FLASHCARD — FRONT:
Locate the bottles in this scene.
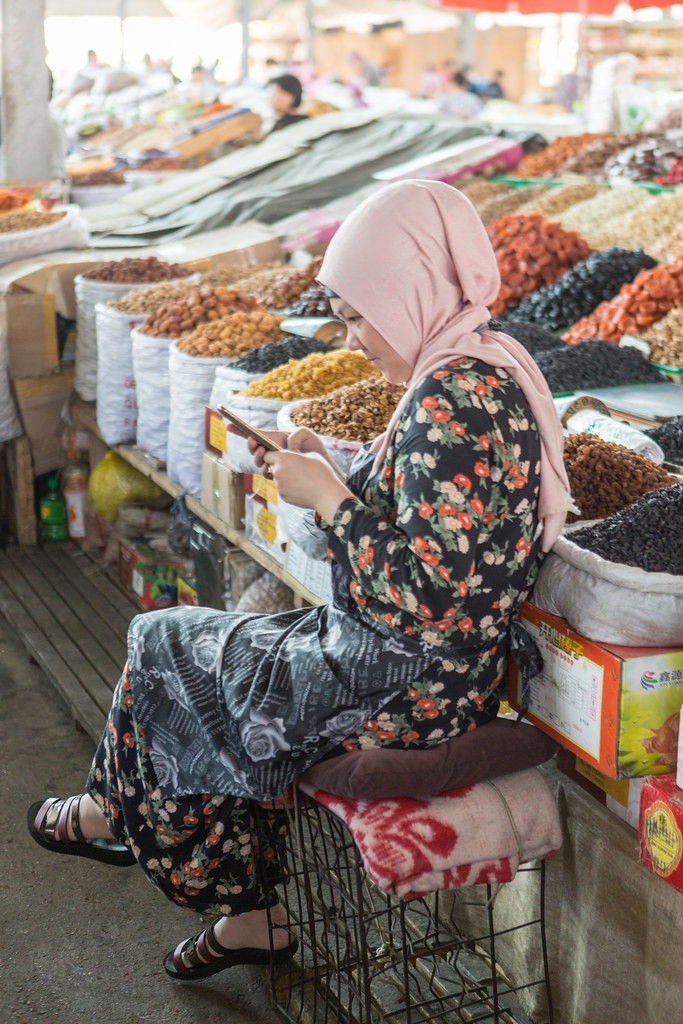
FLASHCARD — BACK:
[40,478,68,543]
[63,451,88,541]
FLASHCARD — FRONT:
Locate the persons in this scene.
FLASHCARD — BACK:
[347,49,389,88]
[24,178,581,980]
[262,73,308,132]
[420,58,505,102]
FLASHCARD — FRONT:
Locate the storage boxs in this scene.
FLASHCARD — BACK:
[6,293,60,379]
[176,575,199,607]
[117,536,183,610]
[10,325,83,477]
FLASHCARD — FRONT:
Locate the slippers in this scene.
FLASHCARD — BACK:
[163,915,299,980]
[27,793,138,867]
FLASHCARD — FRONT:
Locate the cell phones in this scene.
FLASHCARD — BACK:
[217,404,282,453]
